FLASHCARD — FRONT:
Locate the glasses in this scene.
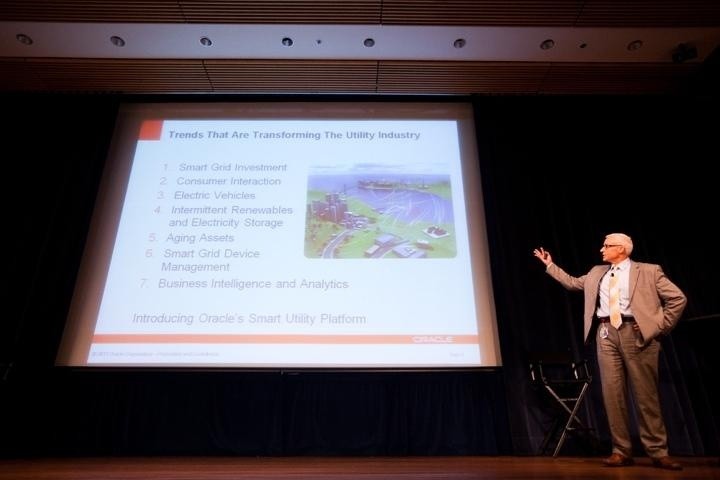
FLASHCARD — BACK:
[602,244,624,249]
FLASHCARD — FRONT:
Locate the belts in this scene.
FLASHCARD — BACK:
[598,318,633,323]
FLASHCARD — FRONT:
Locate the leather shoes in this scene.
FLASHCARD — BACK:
[601,454,633,467]
[653,456,683,470]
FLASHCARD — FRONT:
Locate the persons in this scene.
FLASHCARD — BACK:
[530,230,689,471]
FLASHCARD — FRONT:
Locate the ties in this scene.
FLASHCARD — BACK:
[609,267,623,330]
